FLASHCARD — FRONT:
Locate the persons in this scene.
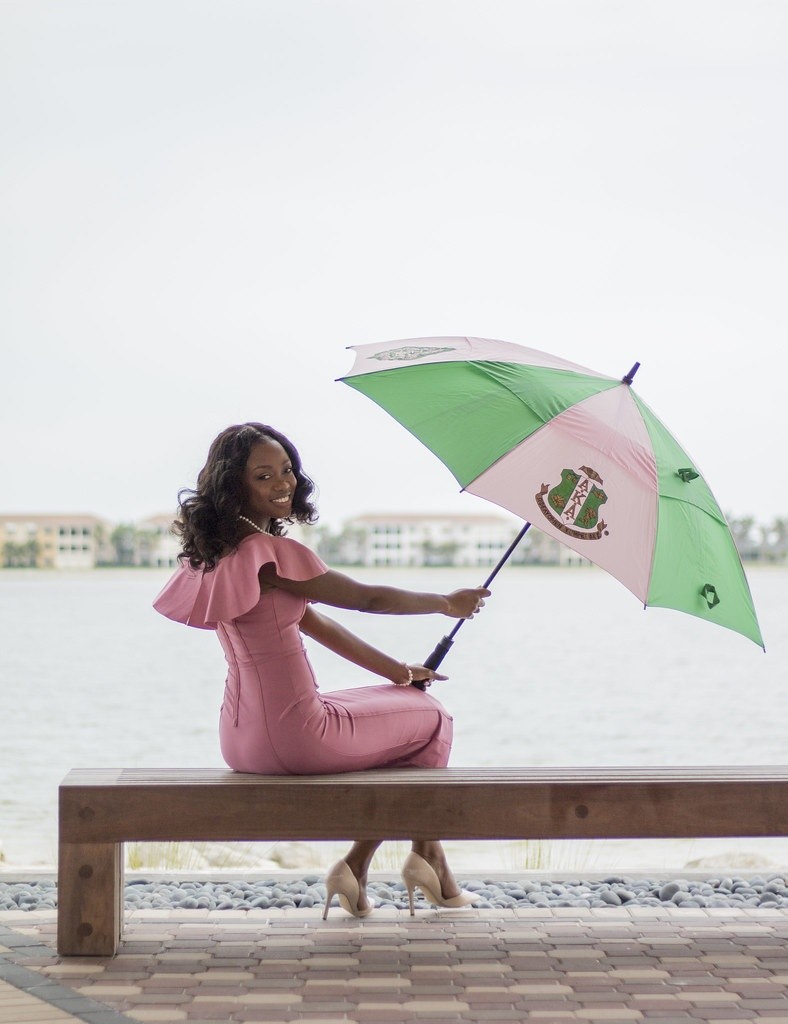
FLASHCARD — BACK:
[151,420,492,925]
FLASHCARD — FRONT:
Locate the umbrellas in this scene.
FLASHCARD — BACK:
[315,334,768,693]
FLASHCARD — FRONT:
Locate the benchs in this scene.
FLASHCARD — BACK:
[57,766,788,957]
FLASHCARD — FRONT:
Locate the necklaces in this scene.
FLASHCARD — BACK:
[239,515,274,536]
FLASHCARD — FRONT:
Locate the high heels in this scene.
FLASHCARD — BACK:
[323,858,376,920]
[402,850,480,916]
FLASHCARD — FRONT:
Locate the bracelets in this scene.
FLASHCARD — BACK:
[393,661,414,686]
